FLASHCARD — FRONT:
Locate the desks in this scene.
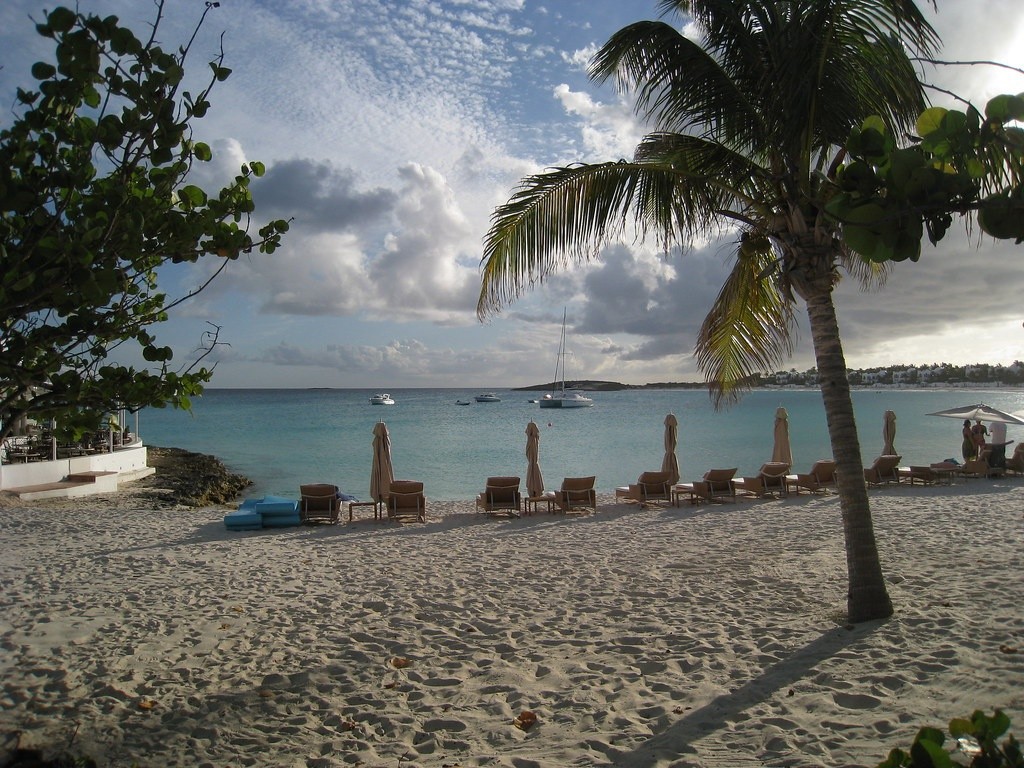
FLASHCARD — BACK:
[898,467,911,485]
[10,453,40,463]
[786,474,798,494]
[731,478,746,496]
[523,494,556,516]
[348,501,377,522]
[672,484,693,508]
[64,448,95,456]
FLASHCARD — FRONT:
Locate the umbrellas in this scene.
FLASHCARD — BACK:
[661,412,680,503]
[772,402,793,492]
[370,420,394,521]
[925,402,1024,425]
[525,417,544,512]
[883,410,897,455]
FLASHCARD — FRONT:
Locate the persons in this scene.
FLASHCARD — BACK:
[962,420,1007,468]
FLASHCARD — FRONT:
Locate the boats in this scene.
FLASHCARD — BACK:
[455,400,471,405]
[371,393,395,405]
[528,399,539,403]
[474,393,502,402]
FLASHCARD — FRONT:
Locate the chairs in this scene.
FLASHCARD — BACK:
[616,472,671,509]
[864,455,902,490]
[547,475,598,513]
[476,476,522,519]
[0,436,51,464]
[387,479,427,523]
[742,463,790,500]
[909,463,931,487]
[796,459,838,496]
[693,468,739,508]
[967,443,1024,479]
[931,460,959,483]
[224,494,301,532]
[300,483,341,526]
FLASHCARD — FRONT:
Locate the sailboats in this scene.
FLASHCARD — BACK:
[540,306,592,409]
